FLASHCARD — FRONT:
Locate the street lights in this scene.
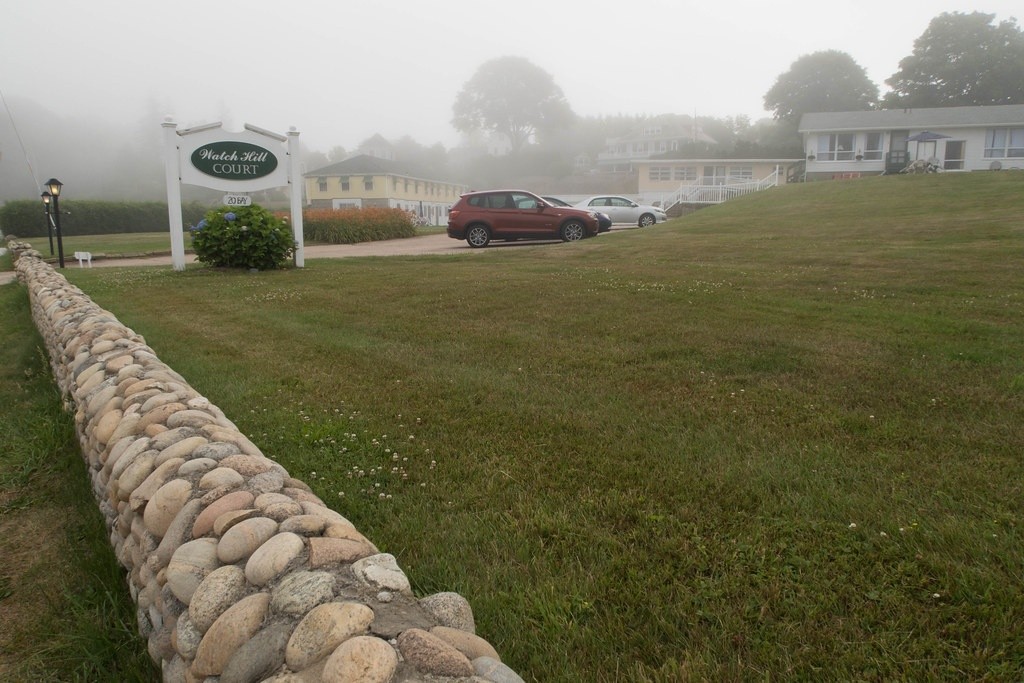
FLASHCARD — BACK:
[43,177,65,268]
[41,191,55,257]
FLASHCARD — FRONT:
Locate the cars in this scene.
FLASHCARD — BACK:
[496,196,613,243]
[571,196,668,227]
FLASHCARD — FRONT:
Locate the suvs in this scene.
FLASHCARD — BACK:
[445,189,600,249]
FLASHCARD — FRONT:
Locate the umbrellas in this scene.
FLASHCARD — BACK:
[906,130,951,162]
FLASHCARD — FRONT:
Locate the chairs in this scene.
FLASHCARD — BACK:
[899,157,944,175]
